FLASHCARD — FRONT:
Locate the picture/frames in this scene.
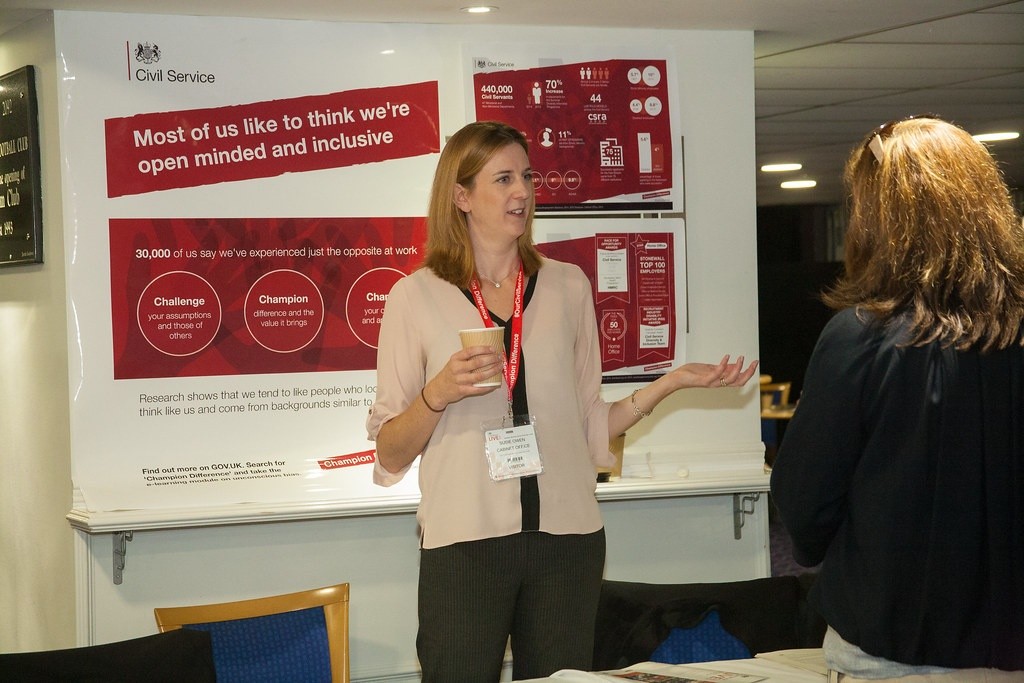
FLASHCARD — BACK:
[0,64,42,268]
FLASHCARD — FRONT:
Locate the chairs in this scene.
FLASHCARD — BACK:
[592,574,827,671]
[155,583,350,683]
[0,629,216,683]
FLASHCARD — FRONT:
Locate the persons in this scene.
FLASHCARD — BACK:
[366,121,759,683]
[771,260,841,450]
[770,115,1023,678]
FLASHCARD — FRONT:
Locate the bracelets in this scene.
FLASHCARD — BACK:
[421,387,447,412]
[632,388,653,417]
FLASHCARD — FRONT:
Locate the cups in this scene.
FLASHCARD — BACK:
[458,326,505,388]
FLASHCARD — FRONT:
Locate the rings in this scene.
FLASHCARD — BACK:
[720,378,726,385]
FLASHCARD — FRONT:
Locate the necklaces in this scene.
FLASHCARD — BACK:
[478,259,519,288]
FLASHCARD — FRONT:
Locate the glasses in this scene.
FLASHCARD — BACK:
[863,114,934,166]
